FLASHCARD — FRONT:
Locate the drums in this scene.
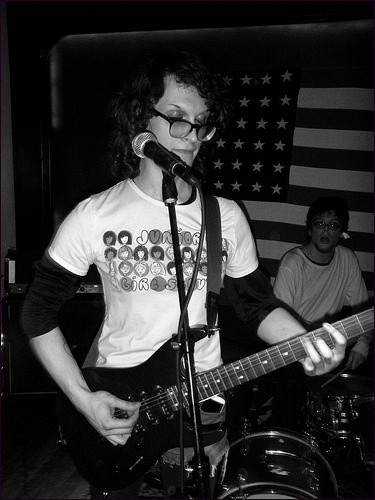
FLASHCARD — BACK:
[302,374,375,439]
[218,432,339,500]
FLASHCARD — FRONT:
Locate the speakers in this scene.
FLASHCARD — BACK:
[6,294,105,395]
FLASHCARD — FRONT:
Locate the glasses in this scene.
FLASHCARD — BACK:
[154,109,216,142]
[312,220,341,230]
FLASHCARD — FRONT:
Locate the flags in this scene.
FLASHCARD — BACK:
[197,65,375,292]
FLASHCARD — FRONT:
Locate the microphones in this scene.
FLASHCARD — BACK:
[131,131,201,188]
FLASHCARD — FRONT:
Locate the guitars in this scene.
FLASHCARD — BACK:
[55,306,375,491]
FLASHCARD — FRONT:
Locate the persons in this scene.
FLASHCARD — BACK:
[21,52,346,499]
[273,197,374,371]
